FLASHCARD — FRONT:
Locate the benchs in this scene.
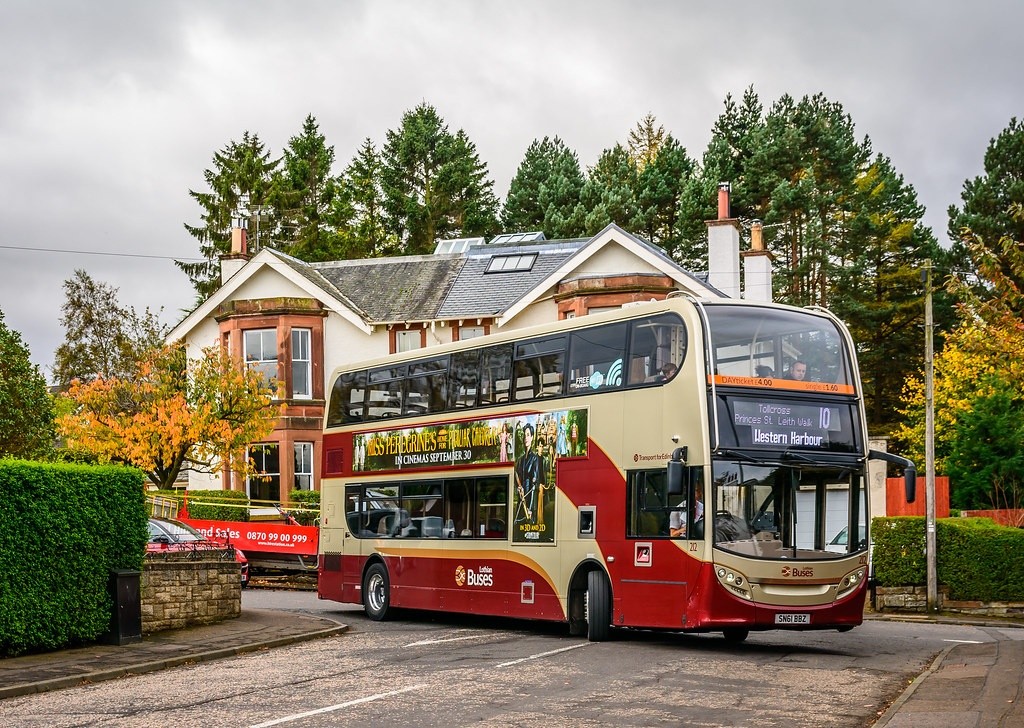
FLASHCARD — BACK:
[347,510,396,537]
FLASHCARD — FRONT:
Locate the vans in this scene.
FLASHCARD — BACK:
[349,490,416,537]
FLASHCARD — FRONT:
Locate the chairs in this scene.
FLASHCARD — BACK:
[535,391,559,399]
[381,412,400,418]
[479,518,505,538]
[450,403,468,409]
[377,515,395,538]
[472,400,493,407]
[422,517,444,538]
[498,397,518,403]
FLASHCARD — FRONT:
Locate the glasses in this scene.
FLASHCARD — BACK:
[695,489,701,492]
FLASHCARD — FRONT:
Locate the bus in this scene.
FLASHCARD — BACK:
[316,296,919,641]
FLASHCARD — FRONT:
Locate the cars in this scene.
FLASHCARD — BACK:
[145,516,249,590]
[458,518,506,537]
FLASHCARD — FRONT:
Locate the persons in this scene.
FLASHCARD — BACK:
[756,365,774,380]
[783,361,806,382]
[516,423,546,533]
[668,479,705,538]
[662,363,677,382]
